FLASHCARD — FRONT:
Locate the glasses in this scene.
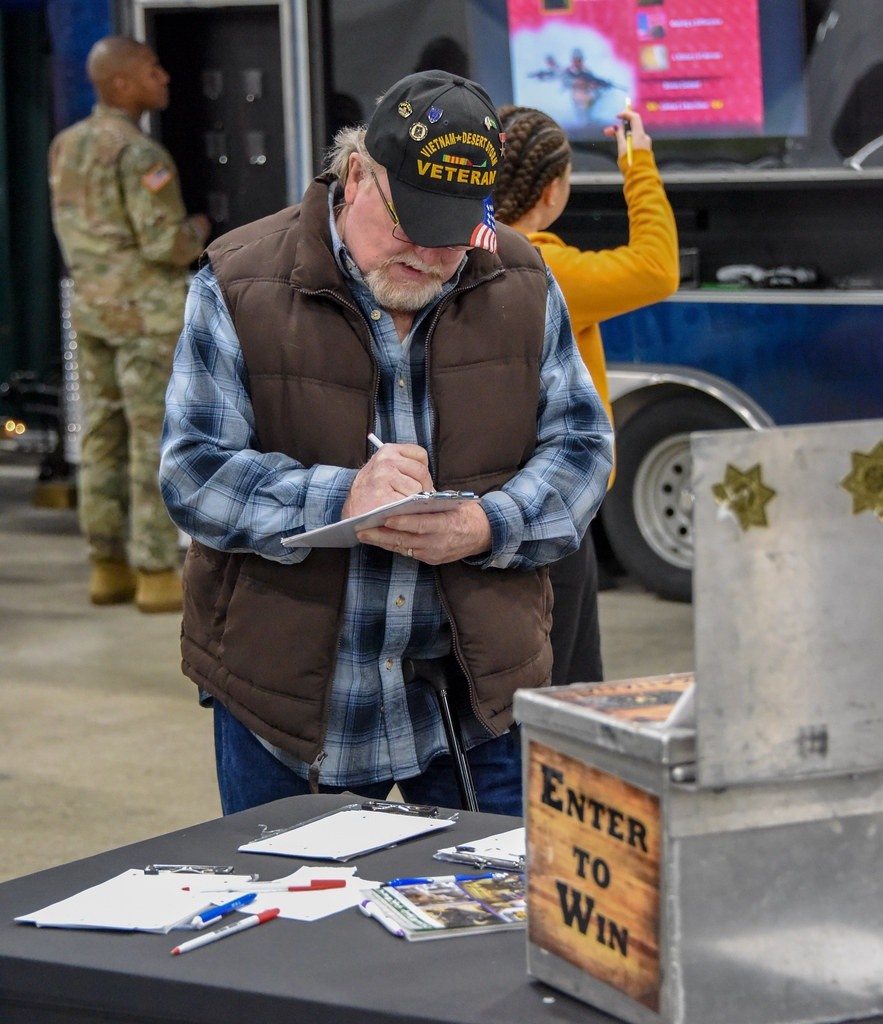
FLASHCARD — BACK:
[369,171,475,252]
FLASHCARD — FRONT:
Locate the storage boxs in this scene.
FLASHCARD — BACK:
[512,413,883,1024]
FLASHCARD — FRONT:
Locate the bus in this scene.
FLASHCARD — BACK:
[0,1,883,609]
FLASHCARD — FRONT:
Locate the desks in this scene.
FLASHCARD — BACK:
[1,793,883,1023]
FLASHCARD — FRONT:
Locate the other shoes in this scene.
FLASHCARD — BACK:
[135,567,184,614]
[89,551,136,605]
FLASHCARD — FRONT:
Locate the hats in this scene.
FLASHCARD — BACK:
[364,69,505,254]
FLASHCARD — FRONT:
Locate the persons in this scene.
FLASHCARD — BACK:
[486,104,679,688]
[157,68,615,819]
[47,35,210,612]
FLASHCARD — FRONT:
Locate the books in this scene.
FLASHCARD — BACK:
[362,871,527,942]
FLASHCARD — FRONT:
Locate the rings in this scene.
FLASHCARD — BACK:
[407,548,413,558]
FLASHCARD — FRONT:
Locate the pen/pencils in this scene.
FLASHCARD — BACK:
[623,96,636,167]
[169,878,347,957]
[358,871,494,937]
[367,431,437,493]
